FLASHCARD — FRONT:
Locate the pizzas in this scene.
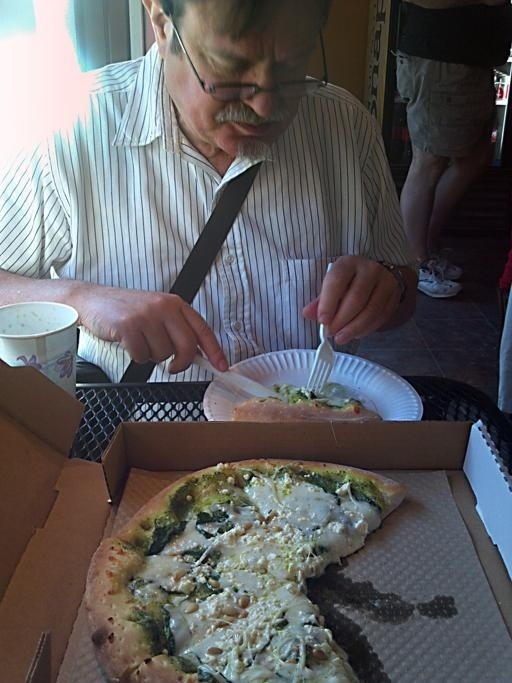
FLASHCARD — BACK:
[230,388,382,423]
[86,458,408,683]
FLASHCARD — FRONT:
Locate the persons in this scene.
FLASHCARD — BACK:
[391,2,510,300]
[2,0,422,421]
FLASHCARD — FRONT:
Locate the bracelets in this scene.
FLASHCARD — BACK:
[378,257,408,307]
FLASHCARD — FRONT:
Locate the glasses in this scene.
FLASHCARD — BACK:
[158,6,328,101]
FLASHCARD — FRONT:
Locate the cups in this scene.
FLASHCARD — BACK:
[0,302,79,399]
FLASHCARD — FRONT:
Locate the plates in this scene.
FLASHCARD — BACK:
[201,348,426,422]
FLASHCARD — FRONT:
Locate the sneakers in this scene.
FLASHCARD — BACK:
[417,262,462,300]
[432,255,463,282]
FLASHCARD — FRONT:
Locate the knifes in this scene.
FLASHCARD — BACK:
[190,351,291,404]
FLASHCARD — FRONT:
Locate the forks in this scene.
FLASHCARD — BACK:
[304,263,335,398]
